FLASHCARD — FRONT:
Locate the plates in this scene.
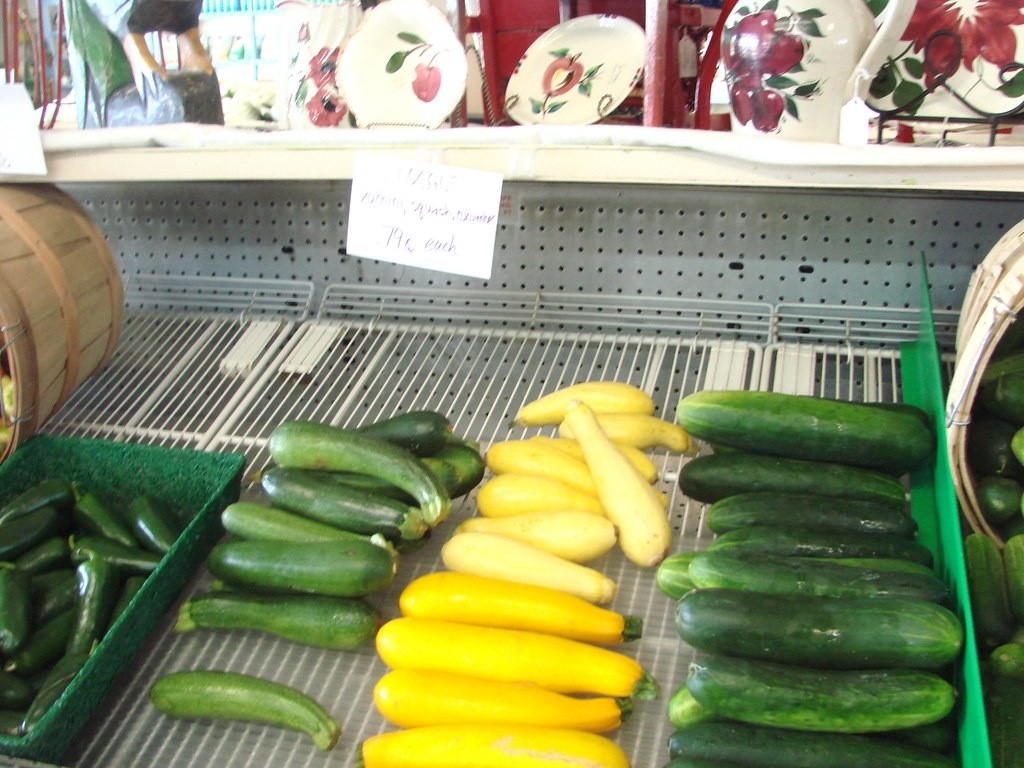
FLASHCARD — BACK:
[505,13,647,127]
[866,0,1024,120]
[335,0,467,128]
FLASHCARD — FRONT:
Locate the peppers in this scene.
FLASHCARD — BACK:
[0,478,180,738]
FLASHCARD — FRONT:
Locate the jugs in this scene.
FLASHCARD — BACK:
[720,0,918,147]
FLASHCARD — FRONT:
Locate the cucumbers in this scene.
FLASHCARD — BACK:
[656,308,1024,768]
